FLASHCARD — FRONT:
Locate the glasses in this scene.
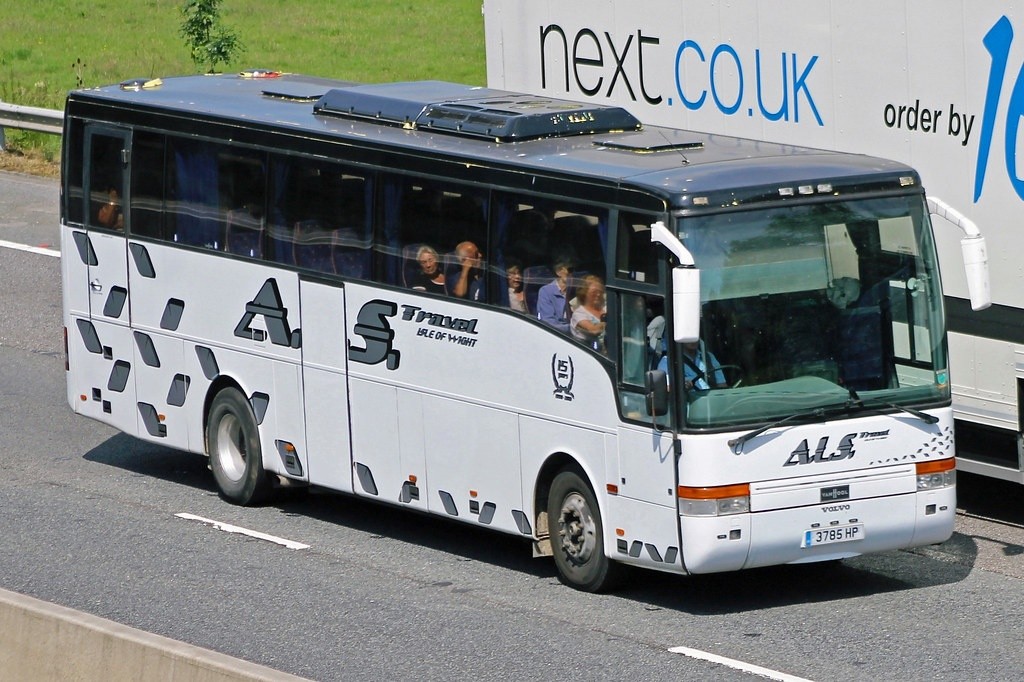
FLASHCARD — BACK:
[508,270,521,275]
[468,250,483,259]
[567,266,582,273]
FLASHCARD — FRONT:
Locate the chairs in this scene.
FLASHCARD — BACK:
[151,183,665,385]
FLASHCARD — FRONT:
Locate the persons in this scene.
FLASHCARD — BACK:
[96,179,124,232]
[653,337,727,394]
[445,239,486,303]
[504,256,528,313]
[406,244,446,294]
[537,253,582,336]
[569,274,608,358]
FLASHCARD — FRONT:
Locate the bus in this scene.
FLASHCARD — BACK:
[61,63,959,591]
